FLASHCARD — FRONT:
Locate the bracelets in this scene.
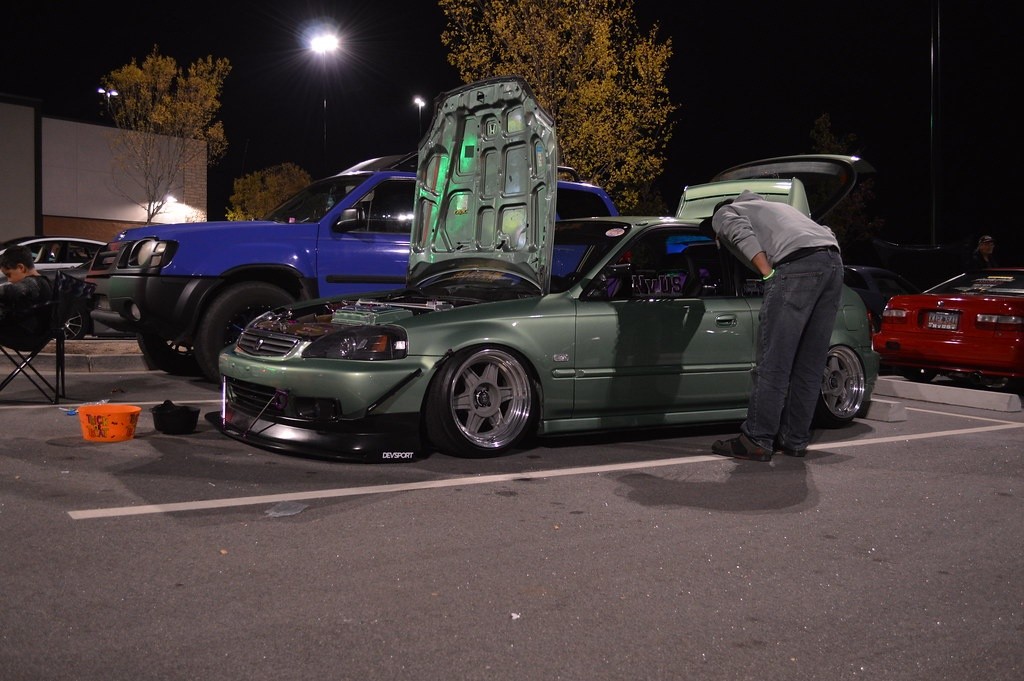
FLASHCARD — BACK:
[763,269,775,280]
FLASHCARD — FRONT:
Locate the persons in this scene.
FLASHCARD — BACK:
[974,235,996,269]
[711,190,844,461]
[0,245,53,309]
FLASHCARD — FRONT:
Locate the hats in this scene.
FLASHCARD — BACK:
[977,235,993,250]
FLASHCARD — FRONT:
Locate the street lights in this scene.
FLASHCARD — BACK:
[310,33,343,177]
[414,96,429,136]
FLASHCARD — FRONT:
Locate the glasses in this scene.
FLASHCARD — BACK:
[983,242,995,247]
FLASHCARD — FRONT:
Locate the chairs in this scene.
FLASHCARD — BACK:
[0,268,99,405]
[640,252,703,300]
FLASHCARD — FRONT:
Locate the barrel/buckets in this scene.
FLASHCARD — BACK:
[148,399,200,435]
[78,404,142,442]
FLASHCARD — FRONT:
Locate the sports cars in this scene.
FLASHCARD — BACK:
[872,268,1024,390]
[214,74,886,458]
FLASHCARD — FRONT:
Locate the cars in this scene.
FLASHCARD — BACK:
[842,263,921,324]
[0,236,108,339]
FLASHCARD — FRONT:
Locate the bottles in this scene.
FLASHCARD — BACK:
[327,198,334,209]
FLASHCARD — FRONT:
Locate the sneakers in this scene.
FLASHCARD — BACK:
[711,433,772,462]
[773,436,806,457]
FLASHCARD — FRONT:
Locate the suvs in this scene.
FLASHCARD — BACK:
[86,149,624,386]
